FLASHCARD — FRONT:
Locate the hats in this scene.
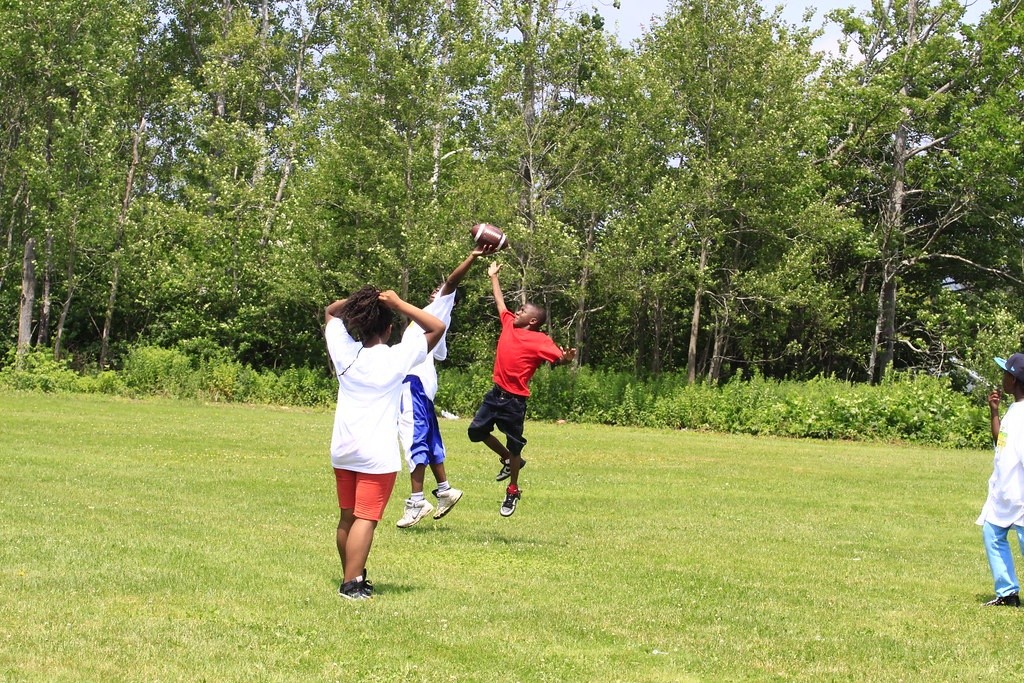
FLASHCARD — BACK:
[994,353,1024,382]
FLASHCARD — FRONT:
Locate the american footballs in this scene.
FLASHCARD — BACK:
[469,222,510,252]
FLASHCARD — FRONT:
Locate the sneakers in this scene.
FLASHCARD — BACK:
[432,487,463,520]
[338,568,377,602]
[496,452,526,481]
[500,488,521,517]
[396,498,435,528]
[981,594,1020,607]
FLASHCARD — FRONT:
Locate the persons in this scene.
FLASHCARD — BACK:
[324,285,447,600]
[981,354,1024,607]
[468,259,577,517]
[396,244,498,528]
